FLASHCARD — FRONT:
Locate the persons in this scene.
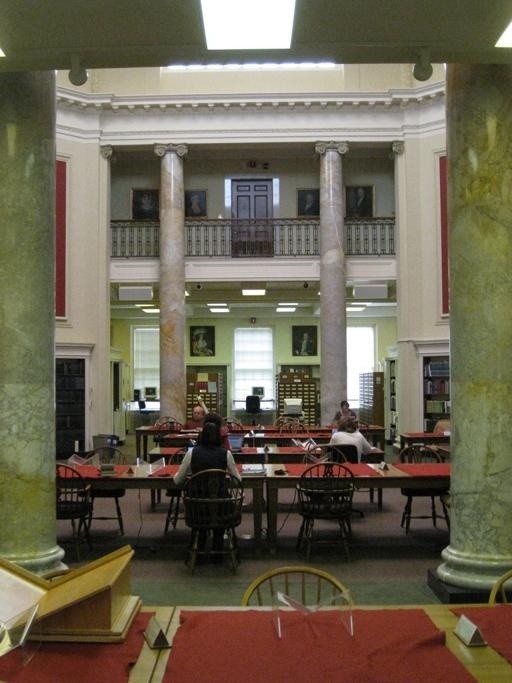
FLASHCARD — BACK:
[191,329,213,355]
[184,405,206,428]
[299,192,319,215]
[171,421,242,566]
[136,190,157,218]
[294,332,312,356]
[328,416,371,465]
[348,186,372,216]
[186,194,205,217]
[202,414,233,452]
[332,400,357,425]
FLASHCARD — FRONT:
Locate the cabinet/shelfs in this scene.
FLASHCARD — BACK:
[383,336,449,444]
[359,373,385,426]
[186,372,223,418]
[55,344,90,460]
[277,378,315,425]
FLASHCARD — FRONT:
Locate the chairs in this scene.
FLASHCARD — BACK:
[241,564,352,606]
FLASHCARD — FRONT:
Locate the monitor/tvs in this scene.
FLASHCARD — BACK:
[283,399,303,415]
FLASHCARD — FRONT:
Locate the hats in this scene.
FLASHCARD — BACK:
[339,416,355,426]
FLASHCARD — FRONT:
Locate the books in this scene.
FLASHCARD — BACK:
[390,360,396,439]
[423,355,451,433]
[275,365,319,424]
[100,462,116,476]
[186,371,223,420]
[240,464,267,478]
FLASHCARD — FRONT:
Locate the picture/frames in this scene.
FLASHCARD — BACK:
[129,186,208,221]
[189,325,216,357]
[291,324,319,357]
[296,185,376,220]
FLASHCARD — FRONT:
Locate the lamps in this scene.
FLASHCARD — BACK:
[412,49,434,81]
[68,54,87,84]
